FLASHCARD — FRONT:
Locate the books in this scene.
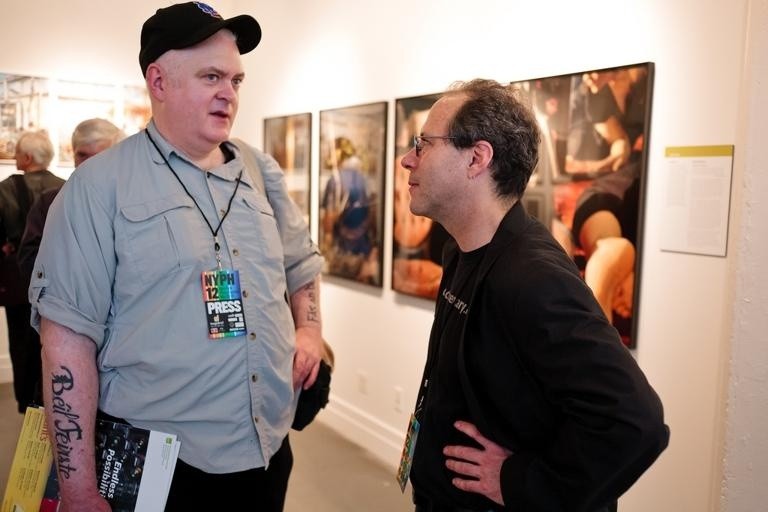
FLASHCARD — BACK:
[0,406,181,511]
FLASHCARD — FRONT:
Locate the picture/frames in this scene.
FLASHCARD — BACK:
[317,100,388,293]
[387,86,475,300]
[261,112,314,246]
[504,60,658,352]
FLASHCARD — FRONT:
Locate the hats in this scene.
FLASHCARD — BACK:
[139,1,261,80]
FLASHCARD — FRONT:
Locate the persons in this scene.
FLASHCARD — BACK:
[16,118,120,285]
[0,129,67,414]
[401,78,670,512]
[27,1,332,512]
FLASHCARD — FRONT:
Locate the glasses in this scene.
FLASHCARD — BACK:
[414,136,460,156]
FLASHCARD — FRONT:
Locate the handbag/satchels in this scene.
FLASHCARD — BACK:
[292,333,335,431]
